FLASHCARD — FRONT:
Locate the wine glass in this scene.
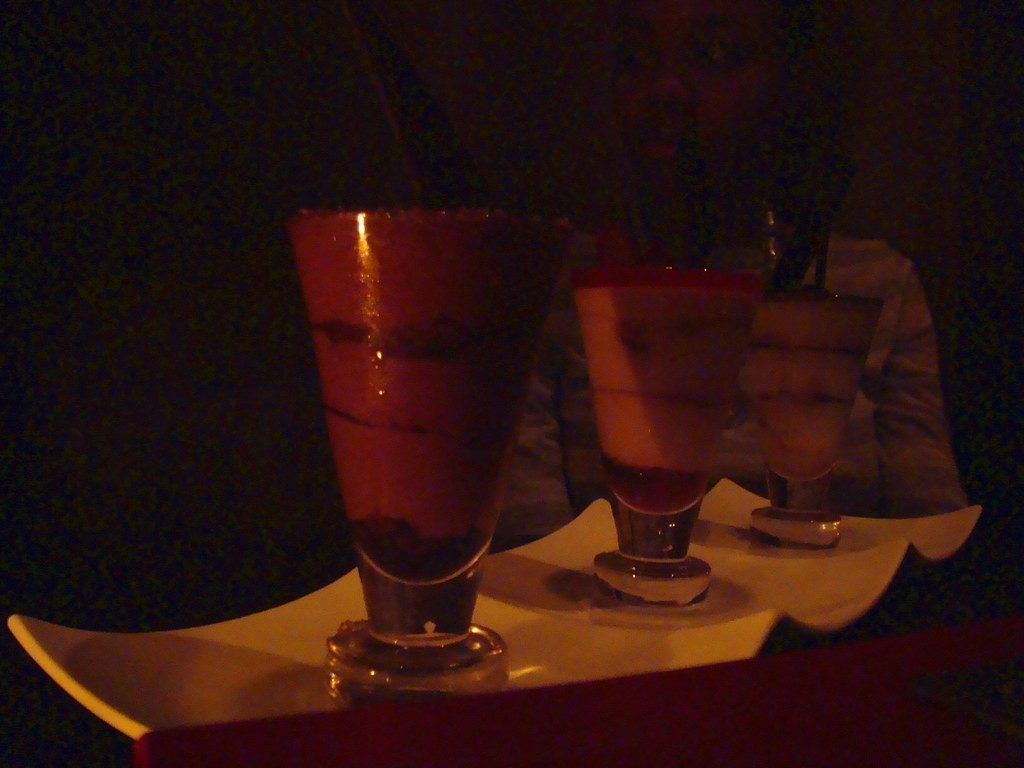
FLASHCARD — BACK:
[746,289,882,544]
[287,203,575,678]
[563,264,757,609]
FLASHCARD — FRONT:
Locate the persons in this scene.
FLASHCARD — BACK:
[494,0,967,546]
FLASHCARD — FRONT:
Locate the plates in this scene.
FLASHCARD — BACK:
[132,614,1024,768]
[4,476,980,740]
